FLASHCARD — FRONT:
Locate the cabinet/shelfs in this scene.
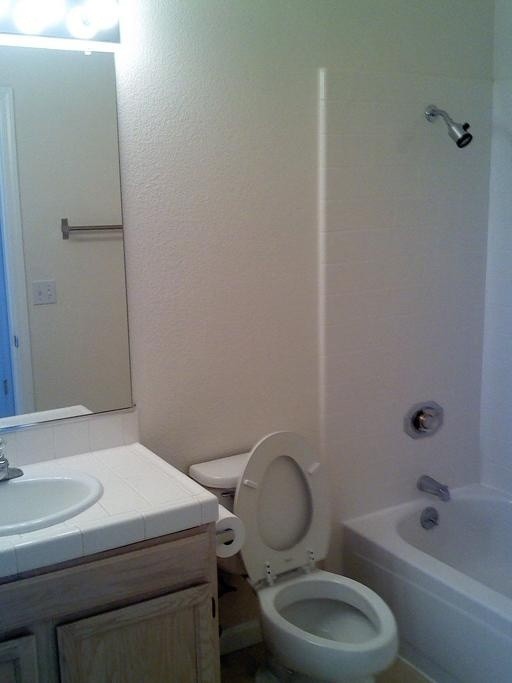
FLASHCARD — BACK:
[0,522,222,683]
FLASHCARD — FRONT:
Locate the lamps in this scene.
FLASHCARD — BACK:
[67,0,117,38]
[8,0,65,33]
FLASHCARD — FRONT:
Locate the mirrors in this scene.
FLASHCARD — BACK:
[0,44,137,436]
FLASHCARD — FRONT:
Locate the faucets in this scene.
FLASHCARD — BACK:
[417,473,453,503]
[1,436,24,483]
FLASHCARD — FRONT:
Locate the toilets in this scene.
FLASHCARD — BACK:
[186,429,399,681]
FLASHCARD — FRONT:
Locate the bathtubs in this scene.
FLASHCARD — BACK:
[342,482,511,680]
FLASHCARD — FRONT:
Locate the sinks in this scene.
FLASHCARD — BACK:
[1,472,105,538]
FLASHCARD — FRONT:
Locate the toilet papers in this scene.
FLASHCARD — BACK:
[213,503,248,561]
